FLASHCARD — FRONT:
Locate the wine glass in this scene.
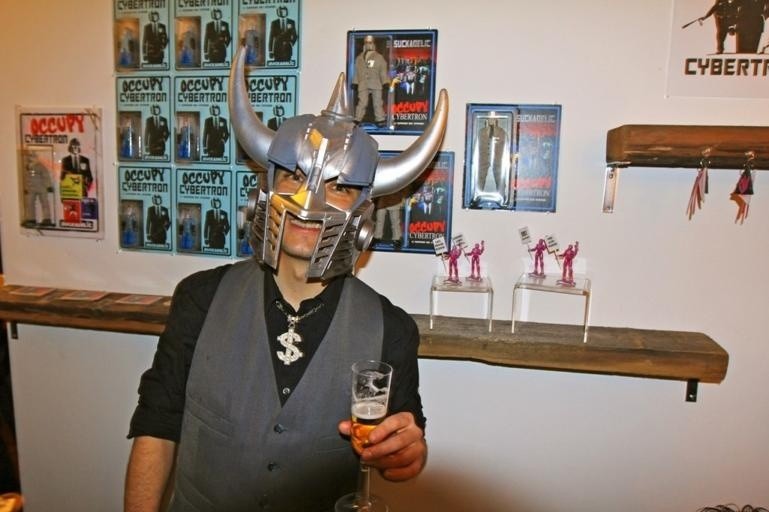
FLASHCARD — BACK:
[333,359,395,512]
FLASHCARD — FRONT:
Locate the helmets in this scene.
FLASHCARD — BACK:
[248,110,382,285]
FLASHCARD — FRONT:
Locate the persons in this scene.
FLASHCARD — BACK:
[59,135,93,200]
[410,180,446,249]
[444,242,463,283]
[464,240,486,282]
[526,238,548,277]
[23,147,56,229]
[351,34,393,128]
[372,189,404,247]
[556,240,582,286]
[124,112,429,511]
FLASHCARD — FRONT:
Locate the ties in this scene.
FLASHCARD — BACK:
[215,209,219,224]
[74,155,79,174]
[216,20,219,33]
[155,116,159,129]
[153,22,157,36]
[156,205,160,217]
[214,117,219,131]
[281,18,286,34]
[277,117,282,130]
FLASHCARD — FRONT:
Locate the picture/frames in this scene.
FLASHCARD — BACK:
[462,103,559,212]
[345,29,436,136]
[367,151,453,255]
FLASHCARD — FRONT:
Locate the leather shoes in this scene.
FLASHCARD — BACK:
[368,238,379,248]
[22,219,51,226]
[393,239,401,250]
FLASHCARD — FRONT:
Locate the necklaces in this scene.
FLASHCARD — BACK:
[271,299,328,366]
